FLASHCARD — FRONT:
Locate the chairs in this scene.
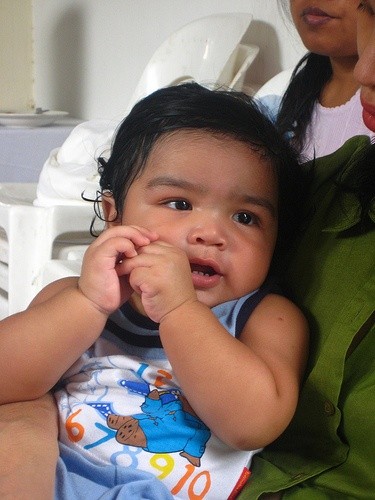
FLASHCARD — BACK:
[0,12,260,318]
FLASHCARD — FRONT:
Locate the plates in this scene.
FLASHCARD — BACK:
[0,111,69,128]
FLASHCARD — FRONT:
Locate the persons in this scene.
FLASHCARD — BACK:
[0,83,308,500]
[241,0,375,500]
[254,0,375,163]
